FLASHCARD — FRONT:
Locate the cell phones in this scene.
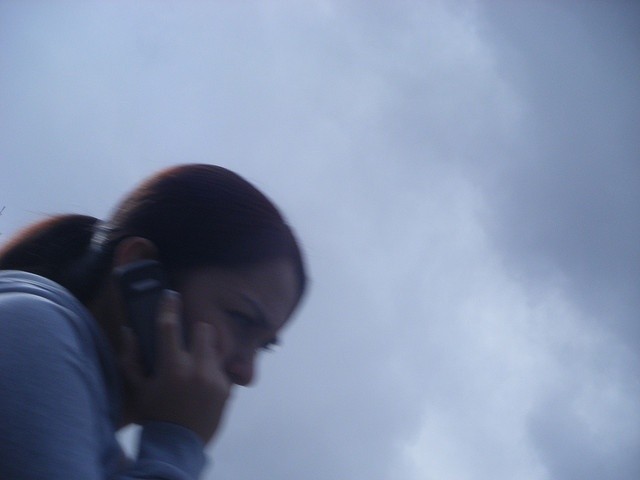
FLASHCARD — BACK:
[110,260,188,380]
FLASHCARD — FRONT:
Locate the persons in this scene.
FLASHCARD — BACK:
[0,162,309,480]
